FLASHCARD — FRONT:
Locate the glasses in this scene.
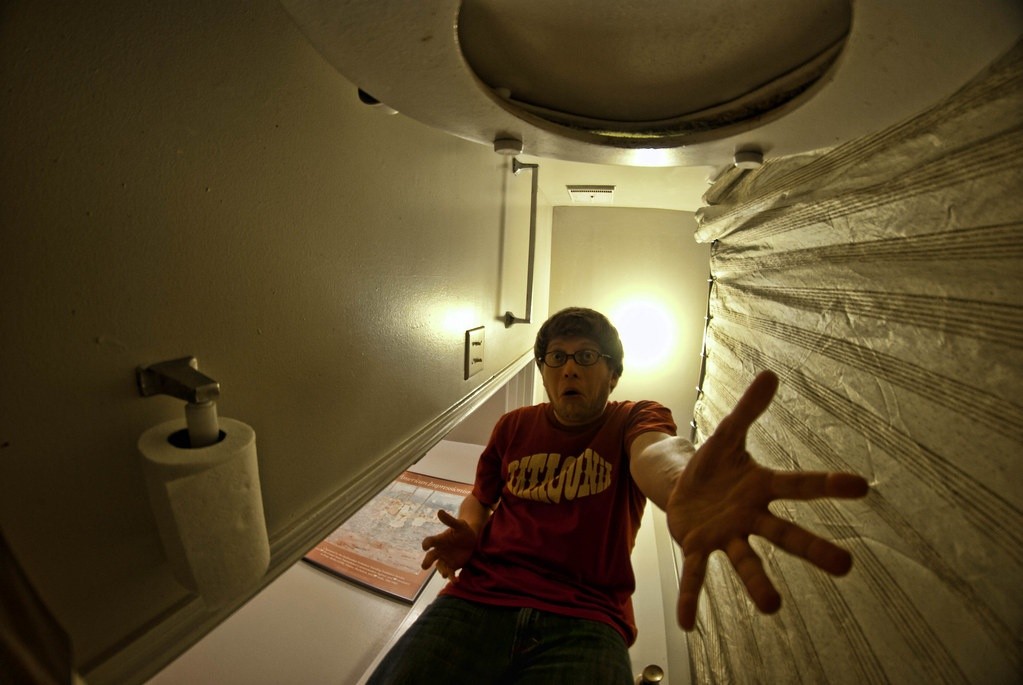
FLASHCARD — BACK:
[538,348,611,368]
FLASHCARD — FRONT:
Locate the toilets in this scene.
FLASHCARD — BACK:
[275,0,1023,170]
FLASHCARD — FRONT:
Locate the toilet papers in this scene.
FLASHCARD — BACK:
[136,415,271,615]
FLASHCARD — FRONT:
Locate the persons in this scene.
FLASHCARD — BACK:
[362,307,869,685]
